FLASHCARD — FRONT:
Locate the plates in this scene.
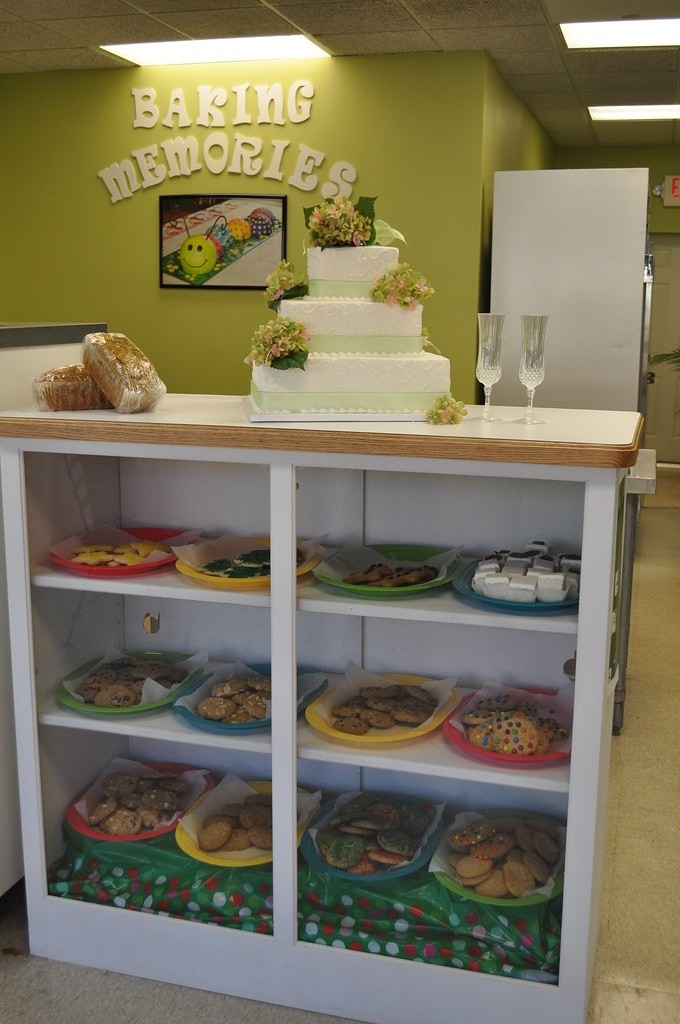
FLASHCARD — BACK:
[300,792,445,881]
[305,673,461,743]
[67,762,213,842]
[432,810,566,906]
[175,537,326,588]
[443,688,571,762]
[175,780,322,867]
[312,543,462,597]
[49,528,201,579]
[452,559,579,612]
[173,664,328,729]
[55,651,204,714]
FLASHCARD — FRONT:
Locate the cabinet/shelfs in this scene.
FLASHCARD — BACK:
[0,393,644,1024]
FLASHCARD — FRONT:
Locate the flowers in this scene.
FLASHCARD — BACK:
[424,391,468,426]
[302,195,407,255]
[262,258,309,314]
[421,326,441,354]
[243,315,311,372]
[367,262,435,313]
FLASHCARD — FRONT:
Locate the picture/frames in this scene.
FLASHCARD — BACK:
[158,193,288,291]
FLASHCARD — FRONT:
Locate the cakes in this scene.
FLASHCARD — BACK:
[245,195,468,426]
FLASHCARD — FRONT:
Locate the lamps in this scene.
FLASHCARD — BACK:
[652,175,680,207]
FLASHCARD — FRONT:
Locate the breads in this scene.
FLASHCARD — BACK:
[81,331,168,415]
[31,362,117,411]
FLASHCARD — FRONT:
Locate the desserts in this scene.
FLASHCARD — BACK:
[470,539,582,604]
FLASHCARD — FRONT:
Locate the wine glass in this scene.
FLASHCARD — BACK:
[472,313,505,423]
[514,314,549,424]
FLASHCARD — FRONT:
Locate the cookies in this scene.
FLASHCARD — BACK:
[188,547,305,582]
[329,682,437,736]
[196,671,271,725]
[316,790,432,875]
[197,792,272,853]
[460,693,569,758]
[341,561,439,588]
[87,769,188,838]
[75,656,190,709]
[446,814,561,898]
[68,539,167,567]
